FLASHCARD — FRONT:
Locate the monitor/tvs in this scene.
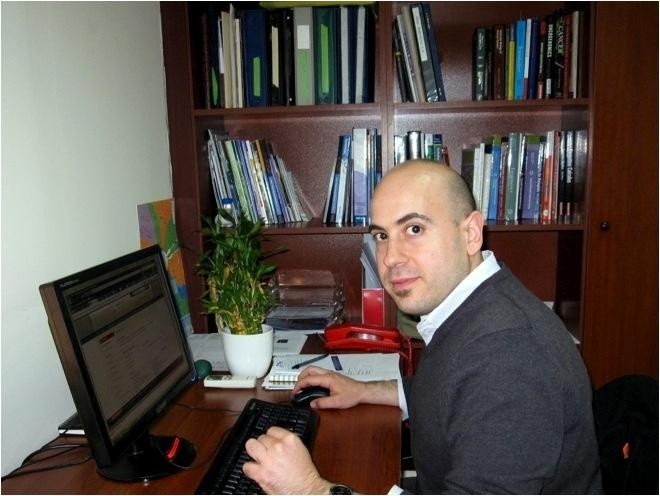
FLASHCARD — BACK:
[39,244,198,483]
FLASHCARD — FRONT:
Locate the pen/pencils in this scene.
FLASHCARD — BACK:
[292,354,329,369]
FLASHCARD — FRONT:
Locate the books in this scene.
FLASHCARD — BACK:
[269,353,400,382]
[472,3,587,100]
[206,2,377,108]
[266,307,338,335]
[392,2,446,102]
[393,128,588,227]
[323,128,383,226]
[205,129,313,224]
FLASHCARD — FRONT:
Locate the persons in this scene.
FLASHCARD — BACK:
[242,161,604,495]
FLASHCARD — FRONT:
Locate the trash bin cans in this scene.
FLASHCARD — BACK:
[324,323,402,352]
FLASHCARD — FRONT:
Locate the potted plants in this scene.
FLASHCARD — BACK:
[192,208,278,375]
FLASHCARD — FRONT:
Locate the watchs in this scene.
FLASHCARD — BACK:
[329,484,353,495]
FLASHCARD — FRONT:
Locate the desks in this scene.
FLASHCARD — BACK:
[0,328,400,494]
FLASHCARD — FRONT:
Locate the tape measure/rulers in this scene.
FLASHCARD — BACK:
[331,356,343,370]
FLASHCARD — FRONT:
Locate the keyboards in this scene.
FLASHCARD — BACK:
[193,397,321,495]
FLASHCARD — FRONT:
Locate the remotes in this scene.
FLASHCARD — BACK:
[203,374,258,390]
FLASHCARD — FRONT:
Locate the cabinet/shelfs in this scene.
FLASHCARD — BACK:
[180,1,591,369]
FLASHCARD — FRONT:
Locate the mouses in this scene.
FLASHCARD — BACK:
[292,386,329,410]
